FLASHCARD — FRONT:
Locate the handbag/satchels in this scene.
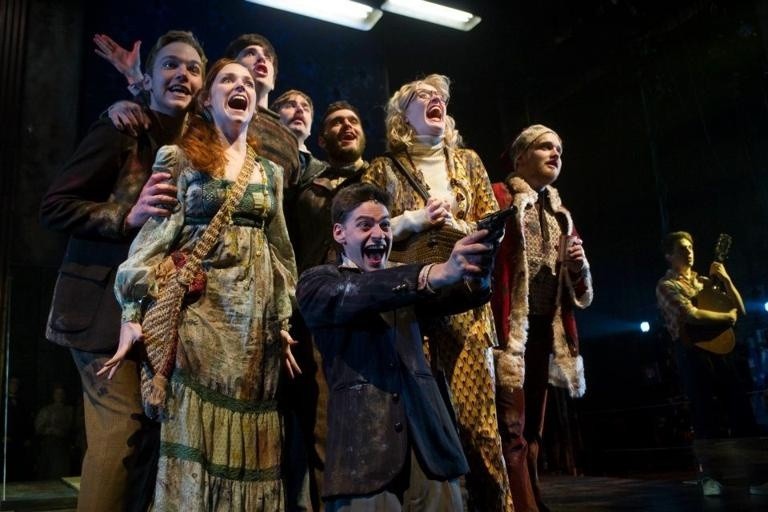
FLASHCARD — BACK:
[136,272,188,412]
[388,223,467,265]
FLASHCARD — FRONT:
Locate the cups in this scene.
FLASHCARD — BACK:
[556,234,576,261]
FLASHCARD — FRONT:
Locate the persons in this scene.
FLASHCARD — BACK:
[653,230,749,496]
[8,28,595,511]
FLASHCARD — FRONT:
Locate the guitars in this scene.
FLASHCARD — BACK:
[681,233,736,355]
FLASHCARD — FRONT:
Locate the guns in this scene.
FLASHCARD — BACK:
[476,206,518,268]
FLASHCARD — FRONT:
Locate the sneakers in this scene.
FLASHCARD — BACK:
[748,482,768,496]
[701,479,728,497]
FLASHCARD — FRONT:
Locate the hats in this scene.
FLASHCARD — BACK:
[509,123,558,172]
